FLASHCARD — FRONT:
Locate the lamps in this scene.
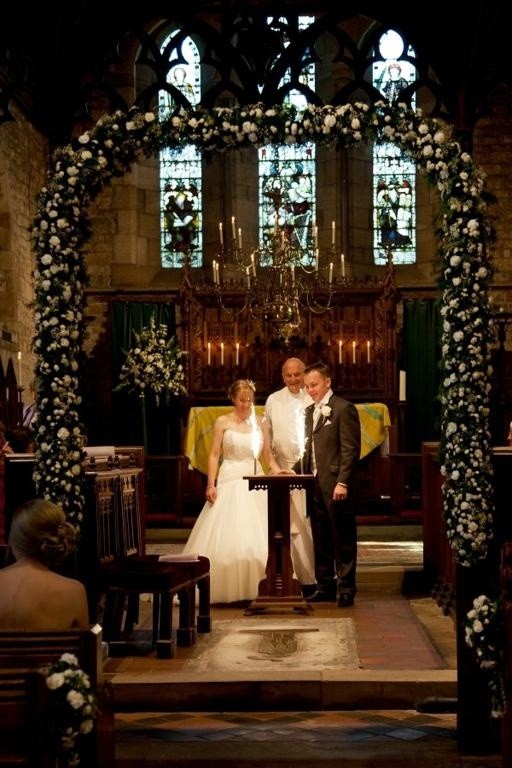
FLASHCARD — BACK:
[213,145,353,347]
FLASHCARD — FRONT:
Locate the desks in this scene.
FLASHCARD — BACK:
[184,403,392,515]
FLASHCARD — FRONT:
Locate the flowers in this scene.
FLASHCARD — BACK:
[465,595,507,719]
[111,315,190,407]
[26,100,495,568]
[38,652,98,767]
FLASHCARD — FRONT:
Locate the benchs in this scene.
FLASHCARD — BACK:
[0,623,116,768]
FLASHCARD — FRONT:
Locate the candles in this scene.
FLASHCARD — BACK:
[353,341,356,364]
[236,343,239,365]
[339,340,342,364]
[208,342,210,365]
[221,343,224,366]
[399,370,406,400]
[18,352,22,387]
[367,341,370,364]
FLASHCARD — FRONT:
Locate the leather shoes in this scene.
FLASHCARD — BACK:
[338,592,354,607]
[305,590,336,602]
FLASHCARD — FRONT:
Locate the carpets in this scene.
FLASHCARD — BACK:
[180,617,362,673]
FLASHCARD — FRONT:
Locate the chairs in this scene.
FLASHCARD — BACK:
[84,472,193,659]
[112,469,212,644]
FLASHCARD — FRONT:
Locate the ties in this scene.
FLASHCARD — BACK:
[310,402,322,479]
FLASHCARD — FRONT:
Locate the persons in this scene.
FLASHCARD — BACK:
[260,358,326,470]
[0,499,106,698]
[280,365,363,610]
[136,377,318,605]
